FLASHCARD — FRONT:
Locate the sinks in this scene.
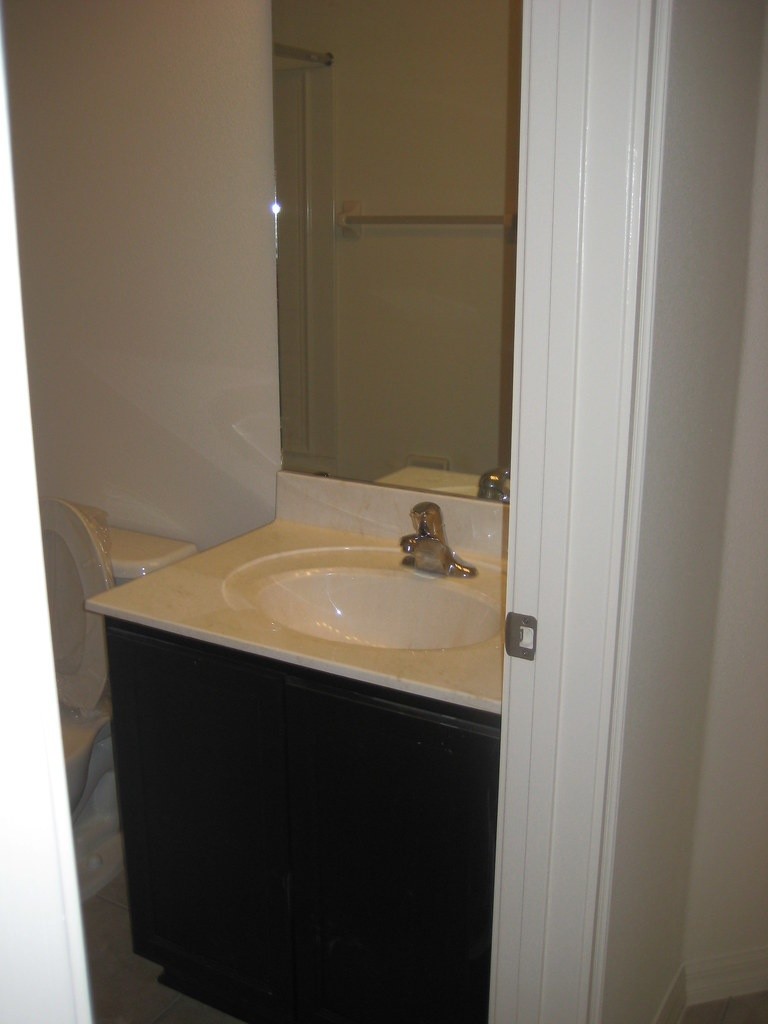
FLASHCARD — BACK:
[437,484,478,499]
[259,566,501,651]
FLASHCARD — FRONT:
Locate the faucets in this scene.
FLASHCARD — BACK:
[479,468,511,504]
[400,501,477,579]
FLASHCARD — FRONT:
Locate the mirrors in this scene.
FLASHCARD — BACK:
[267,0,523,506]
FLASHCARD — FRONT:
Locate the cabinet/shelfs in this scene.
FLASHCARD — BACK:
[104,618,503,1023]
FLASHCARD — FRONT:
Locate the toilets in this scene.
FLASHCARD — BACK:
[37,498,197,903]
